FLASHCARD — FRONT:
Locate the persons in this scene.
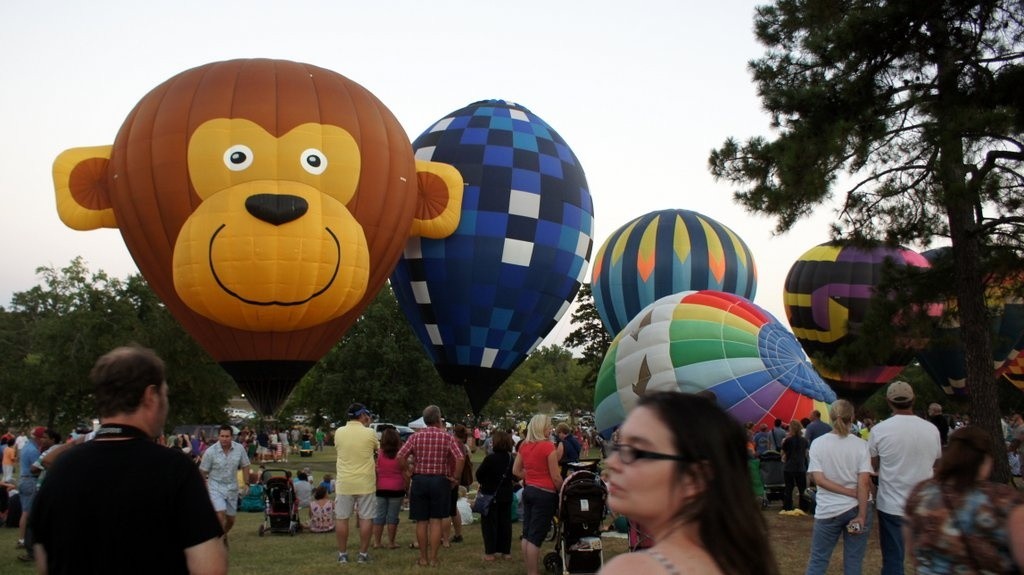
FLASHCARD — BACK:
[806,399,874,575]
[868,380,941,575]
[0,393,101,563]
[595,391,779,575]
[924,401,1024,475]
[26,347,228,575]
[903,425,1024,575]
[695,389,873,513]
[157,404,640,575]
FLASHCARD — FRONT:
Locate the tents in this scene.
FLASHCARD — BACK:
[408,415,452,430]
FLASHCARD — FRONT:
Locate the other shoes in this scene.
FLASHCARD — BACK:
[337,555,348,563]
[358,553,374,563]
[17,542,26,548]
[779,510,794,515]
[794,509,808,516]
[450,535,463,544]
[18,555,33,560]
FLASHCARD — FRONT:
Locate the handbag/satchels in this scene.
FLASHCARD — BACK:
[302,433,309,441]
[460,447,474,485]
[776,447,782,452]
[472,490,494,516]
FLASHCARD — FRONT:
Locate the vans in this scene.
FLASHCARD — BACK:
[368,422,400,441]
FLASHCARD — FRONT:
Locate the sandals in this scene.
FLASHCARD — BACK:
[410,543,420,550]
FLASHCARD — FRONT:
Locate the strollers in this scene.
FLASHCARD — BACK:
[543,467,609,575]
[258,468,303,537]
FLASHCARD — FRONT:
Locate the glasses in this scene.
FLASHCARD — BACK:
[607,440,694,464]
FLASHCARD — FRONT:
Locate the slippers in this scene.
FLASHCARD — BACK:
[416,559,429,565]
[431,559,440,566]
[494,553,512,560]
[482,554,495,561]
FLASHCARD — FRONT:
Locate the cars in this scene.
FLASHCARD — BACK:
[395,425,415,441]
[551,414,570,424]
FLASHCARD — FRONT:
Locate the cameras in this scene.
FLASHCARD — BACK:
[846,523,861,534]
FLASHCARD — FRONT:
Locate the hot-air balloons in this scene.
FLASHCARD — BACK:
[592,208,759,340]
[388,95,595,435]
[592,290,839,436]
[783,237,949,416]
[913,245,1023,421]
[53,57,470,437]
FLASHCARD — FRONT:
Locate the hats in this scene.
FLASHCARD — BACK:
[887,381,914,403]
[32,426,48,437]
[347,403,369,415]
